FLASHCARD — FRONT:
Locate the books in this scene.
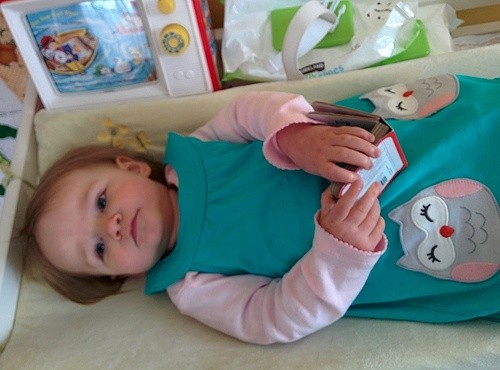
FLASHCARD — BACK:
[306,101,407,202]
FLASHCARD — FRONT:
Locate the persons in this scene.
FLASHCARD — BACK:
[23,71,500,345]
